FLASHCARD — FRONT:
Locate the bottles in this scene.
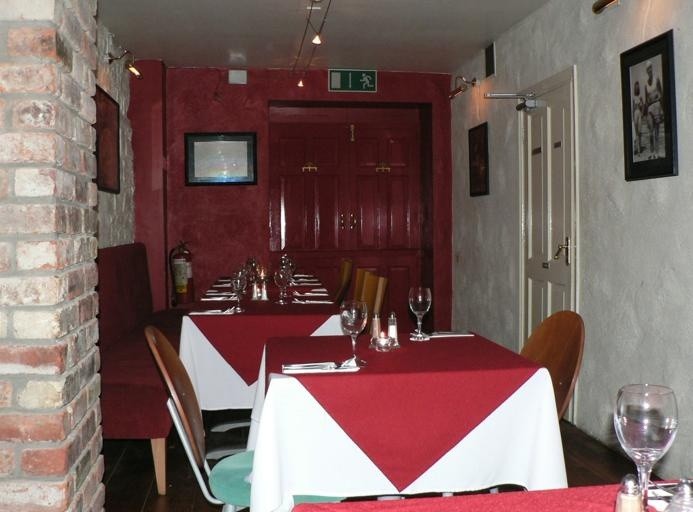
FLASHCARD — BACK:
[614,472,692,512]
[369,311,401,352]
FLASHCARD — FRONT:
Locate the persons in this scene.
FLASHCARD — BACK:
[643,60,664,159]
[632,81,644,153]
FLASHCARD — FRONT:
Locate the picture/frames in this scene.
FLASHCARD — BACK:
[468,120,490,197]
[91,84,120,194]
[620,28,678,182]
[184,131,258,187]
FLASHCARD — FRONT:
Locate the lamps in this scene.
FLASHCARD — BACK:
[105,47,144,81]
[447,75,480,101]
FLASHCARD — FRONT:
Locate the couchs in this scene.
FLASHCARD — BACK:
[94,241,188,497]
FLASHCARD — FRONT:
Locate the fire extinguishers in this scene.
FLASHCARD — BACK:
[169,239,194,306]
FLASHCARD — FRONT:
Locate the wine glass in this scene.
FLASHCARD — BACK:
[611,382,679,510]
[339,300,373,369]
[231,253,296,315]
[407,286,432,341]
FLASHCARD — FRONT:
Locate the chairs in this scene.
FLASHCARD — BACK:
[143,323,349,512]
[440,309,586,498]
[333,258,389,334]
